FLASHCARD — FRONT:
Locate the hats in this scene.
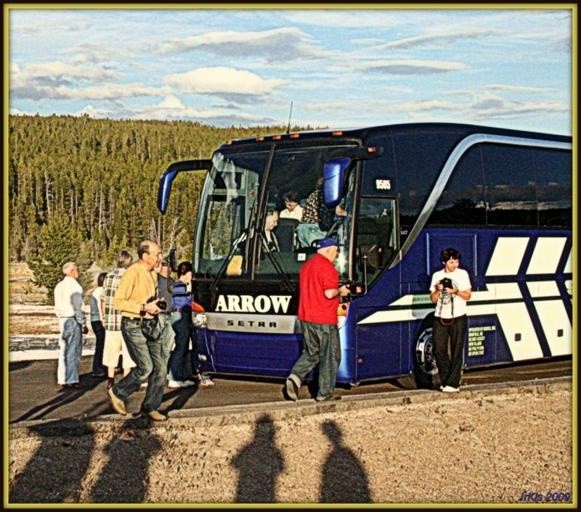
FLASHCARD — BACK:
[316,239,344,249]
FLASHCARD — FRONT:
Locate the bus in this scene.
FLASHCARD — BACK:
[155,121,571,392]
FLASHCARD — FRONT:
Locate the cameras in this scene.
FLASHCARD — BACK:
[339,279,365,299]
[147,295,167,311]
[439,277,453,295]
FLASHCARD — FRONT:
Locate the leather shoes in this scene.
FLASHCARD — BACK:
[72,369,168,421]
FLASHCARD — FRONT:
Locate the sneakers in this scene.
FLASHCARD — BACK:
[440,382,460,392]
[166,373,342,404]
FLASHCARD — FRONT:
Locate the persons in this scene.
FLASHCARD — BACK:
[430,247,472,394]
[54,179,355,419]
